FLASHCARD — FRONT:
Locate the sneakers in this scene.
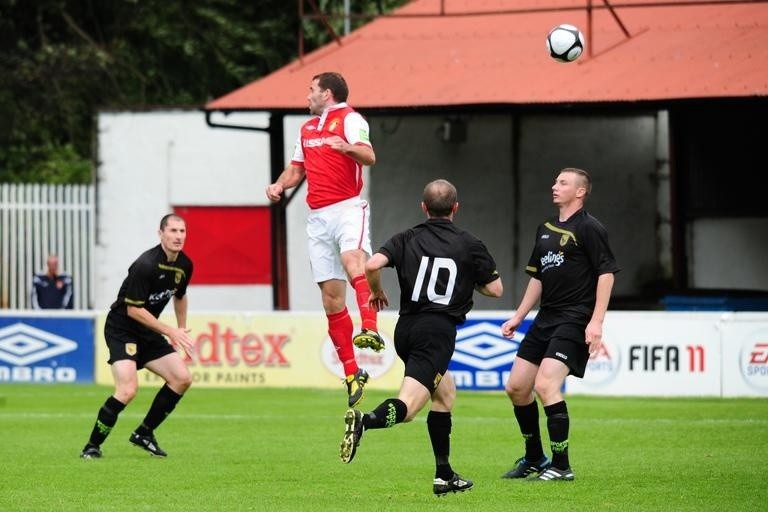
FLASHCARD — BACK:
[352,327,386,353]
[80,447,102,458]
[128,432,167,457]
[529,466,574,481]
[434,472,474,499]
[500,456,551,479]
[340,410,364,463]
[341,368,371,408]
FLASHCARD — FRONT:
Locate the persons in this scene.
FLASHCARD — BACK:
[30,257,74,310]
[339,178,504,495]
[266,72,385,411]
[80,214,195,459]
[499,168,623,481]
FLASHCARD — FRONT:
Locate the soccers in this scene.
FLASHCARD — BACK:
[547,24,583,62]
